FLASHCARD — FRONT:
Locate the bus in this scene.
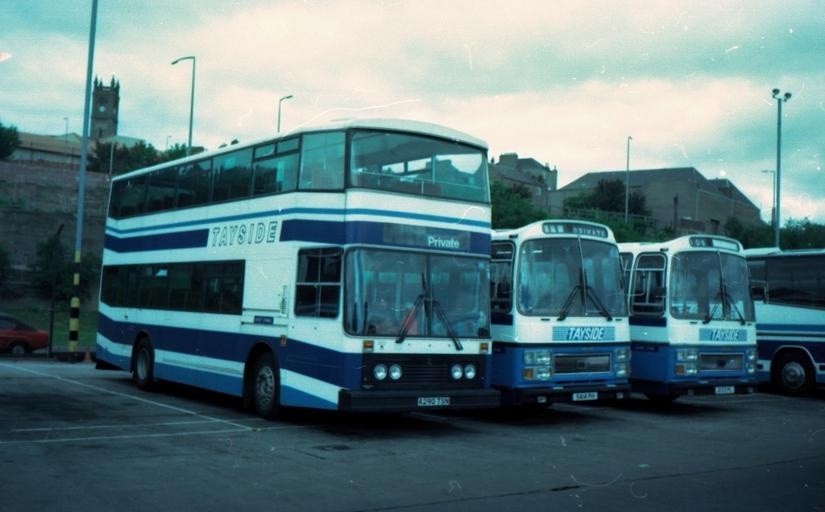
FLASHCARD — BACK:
[745,246,825,395]
[97,118,500,419]
[492,219,633,404]
[618,233,758,404]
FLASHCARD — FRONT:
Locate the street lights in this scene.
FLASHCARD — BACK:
[762,169,776,226]
[276,94,290,134]
[772,88,792,246]
[625,136,635,224]
[171,56,195,153]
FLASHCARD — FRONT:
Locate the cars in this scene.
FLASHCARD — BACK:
[0,315,52,357]
